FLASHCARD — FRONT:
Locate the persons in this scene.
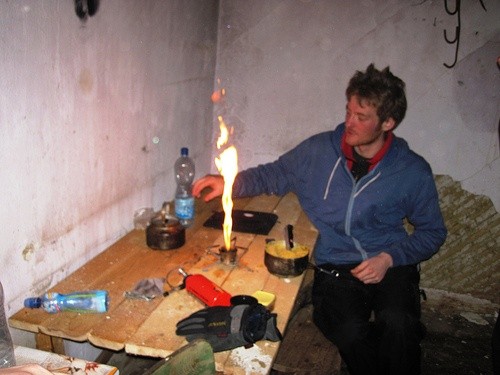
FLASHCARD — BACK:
[192,63,448,375]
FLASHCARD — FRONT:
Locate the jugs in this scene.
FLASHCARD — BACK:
[146,202,185,251]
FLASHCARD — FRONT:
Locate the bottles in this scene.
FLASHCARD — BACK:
[174,148,195,228]
[24,290,108,313]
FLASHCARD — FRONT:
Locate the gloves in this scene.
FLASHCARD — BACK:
[176,303,283,353]
